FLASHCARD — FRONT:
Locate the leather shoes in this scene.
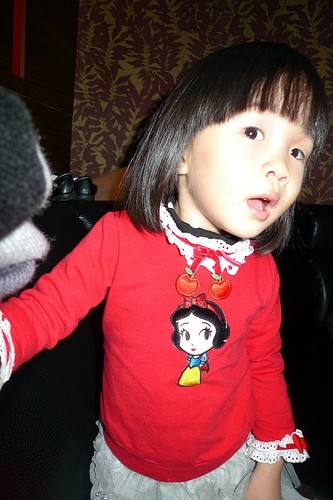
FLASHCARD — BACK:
[75,175,98,200]
[49,173,77,203]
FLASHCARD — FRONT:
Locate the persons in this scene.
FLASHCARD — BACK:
[0,39,331,500]
